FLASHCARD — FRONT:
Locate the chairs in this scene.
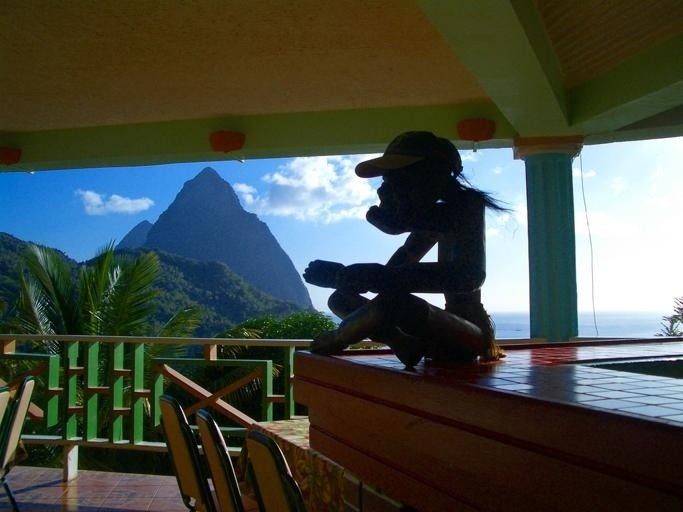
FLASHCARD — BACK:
[0,386,10,427]
[195,408,259,512]
[0,374,37,512]
[245,427,306,512]
[156,395,221,512]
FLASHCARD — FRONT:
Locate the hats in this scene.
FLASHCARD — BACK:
[354,129,462,177]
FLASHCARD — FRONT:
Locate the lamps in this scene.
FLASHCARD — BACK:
[457,117,495,141]
[209,131,245,153]
[0,146,22,165]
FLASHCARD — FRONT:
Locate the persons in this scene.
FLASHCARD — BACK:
[303,131,513,369]
